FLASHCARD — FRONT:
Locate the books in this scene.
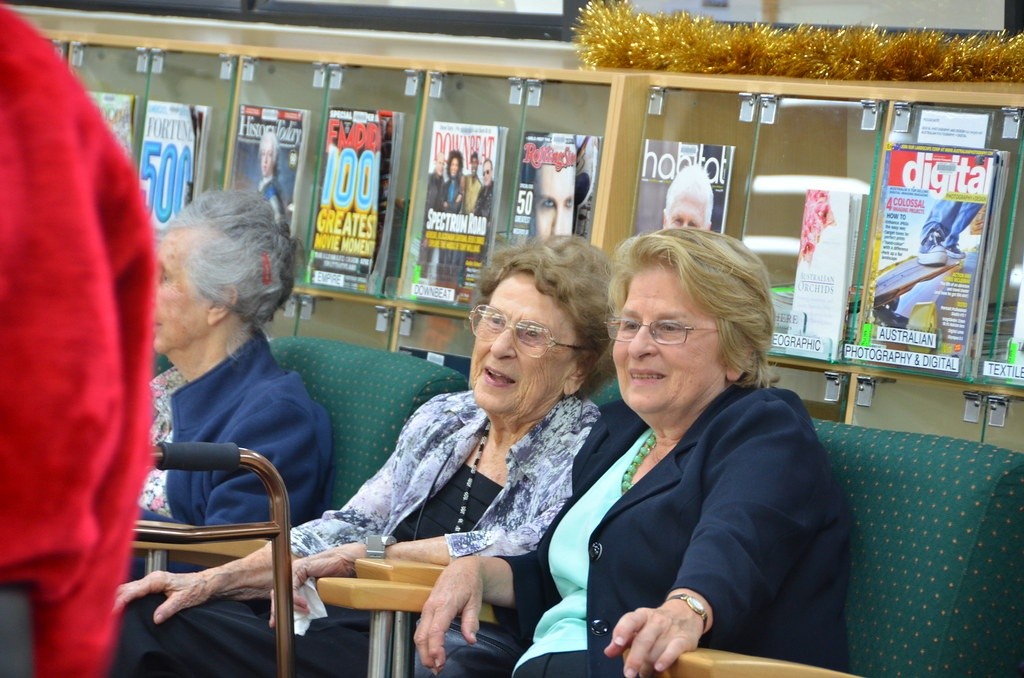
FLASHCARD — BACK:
[306,107,404,296]
[140,99,212,227]
[412,122,508,304]
[229,104,311,237]
[86,92,139,160]
[511,131,603,243]
[866,142,1010,380]
[790,188,865,359]
[635,138,736,235]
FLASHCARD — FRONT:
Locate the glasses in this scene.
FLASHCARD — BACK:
[482,169,493,176]
[468,304,596,359]
[603,317,718,345]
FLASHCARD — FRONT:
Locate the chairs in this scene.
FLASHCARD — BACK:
[317,416,1024,678]
[354,375,621,678]
[134,334,470,574]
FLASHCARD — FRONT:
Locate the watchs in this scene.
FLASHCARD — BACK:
[667,594,707,631]
[365,535,397,559]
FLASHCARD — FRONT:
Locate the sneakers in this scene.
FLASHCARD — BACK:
[945,243,966,259]
[918,228,947,266]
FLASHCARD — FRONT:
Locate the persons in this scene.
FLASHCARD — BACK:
[0,0,154,678]
[528,133,576,237]
[663,166,714,230]
[256,132,285,225]
[108,234,612,678]
[918,199,983,265]
[417,150,495,285]
[126,190,333,579]
[414,227,852,678]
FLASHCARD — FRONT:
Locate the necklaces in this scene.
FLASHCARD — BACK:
[622,433,657,491]
[455,422,490,534]
